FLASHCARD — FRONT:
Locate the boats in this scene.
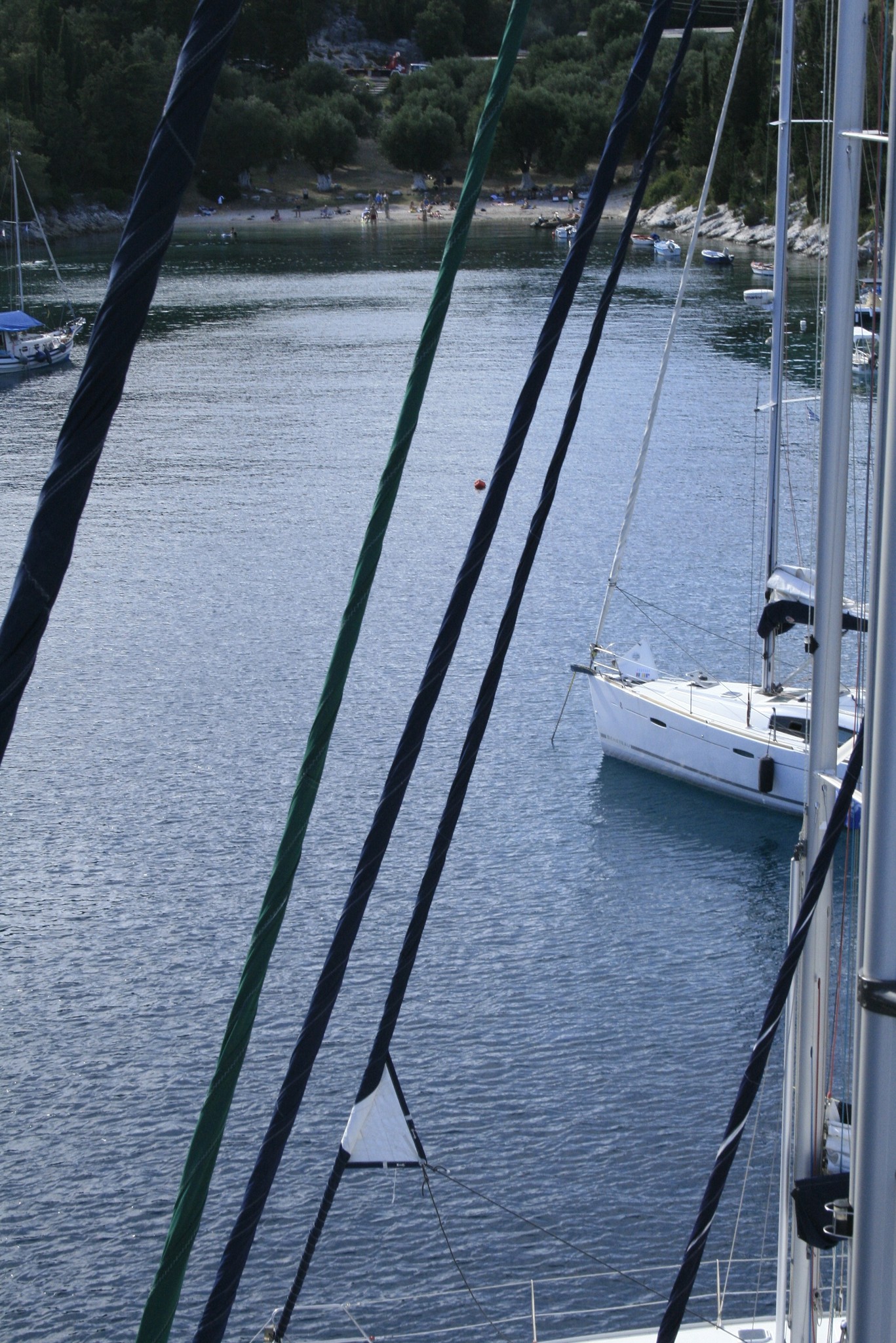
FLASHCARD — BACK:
[529,213,550,228]
[701,246,735,264]
[555,223,577,240]
[540,213,580,229]
[653,237,681,257]
[750,260,789,276]
[743,288,774,305]
[630,233,660,246]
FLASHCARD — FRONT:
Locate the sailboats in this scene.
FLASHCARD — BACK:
[0,144,89,378]
[568,0,869,835]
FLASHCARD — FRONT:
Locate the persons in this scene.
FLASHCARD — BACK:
[578,199,585,215]
[295,197,301,218]
[449,199,456,210]
[303,186,309,206]
[273,209,280,222]
[504,182,544,209]
[230,227,236,235]
[409,194,443,222]
[567,190,574,211]
[322,205,344,219]
[217,195,225,211]
[362,189,390,225]
